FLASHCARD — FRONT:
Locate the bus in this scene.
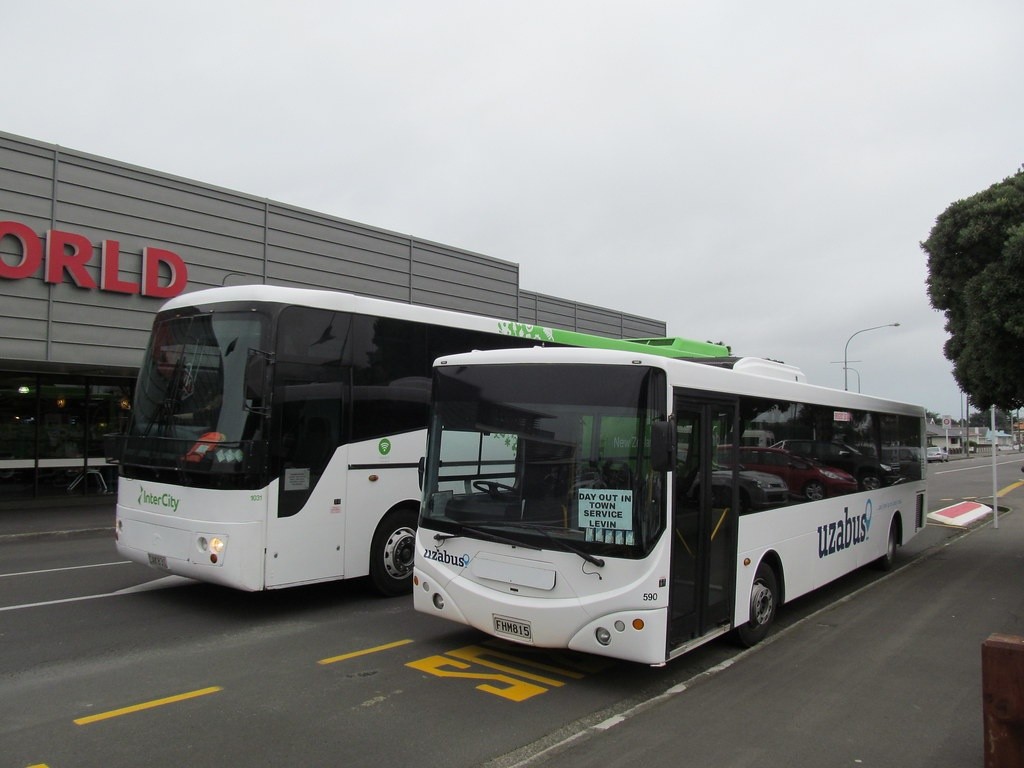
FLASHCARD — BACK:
[410,347,928,670]
[113,283,806,604]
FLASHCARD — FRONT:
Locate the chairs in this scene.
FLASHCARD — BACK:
[602,459,630,490]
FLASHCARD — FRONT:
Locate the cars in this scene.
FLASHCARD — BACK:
[927,446,951,463]
[718,446,858,501]
[872,445,921,480]
[770,440,899,493]
[711,462,788,509]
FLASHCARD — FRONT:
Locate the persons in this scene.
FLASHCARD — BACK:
[542,465,564,525]
[167,391,223,427]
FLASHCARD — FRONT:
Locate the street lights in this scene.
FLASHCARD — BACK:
[843,322,901,392]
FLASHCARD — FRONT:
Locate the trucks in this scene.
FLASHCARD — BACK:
[677,425,776,466]
